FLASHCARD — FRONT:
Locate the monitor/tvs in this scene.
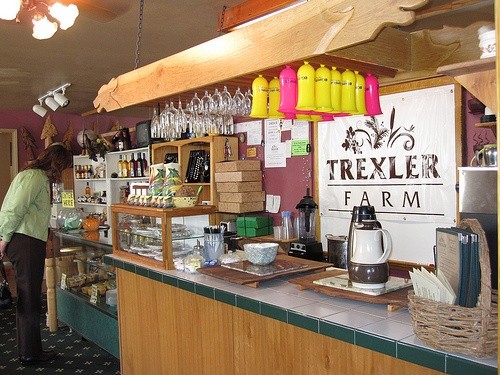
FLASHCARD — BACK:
[136,120,158,148]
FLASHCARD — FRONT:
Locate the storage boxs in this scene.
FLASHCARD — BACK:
[236,215,274,238]
[214,159,266,213]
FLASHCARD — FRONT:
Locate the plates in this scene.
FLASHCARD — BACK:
[123,244,192,262]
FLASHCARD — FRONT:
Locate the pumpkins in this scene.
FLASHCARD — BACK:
[82,216,99,232]
[83,230,100,240]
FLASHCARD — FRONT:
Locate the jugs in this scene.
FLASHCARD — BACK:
[345,205,393,285]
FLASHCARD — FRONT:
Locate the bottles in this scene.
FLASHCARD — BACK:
[85,182,91,194]
[117,151,148,177]
[125,182,130,198]
[118,128,125,151]
[101,190,106,204]
[280,211,293,240]
[74,164,92,179]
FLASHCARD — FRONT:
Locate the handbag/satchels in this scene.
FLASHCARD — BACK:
[0,259,12,310]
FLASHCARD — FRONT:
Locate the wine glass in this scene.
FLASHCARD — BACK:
[149,81,253,141]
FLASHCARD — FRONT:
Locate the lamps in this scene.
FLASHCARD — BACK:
[0,0,79,40]
[32,83,72,118]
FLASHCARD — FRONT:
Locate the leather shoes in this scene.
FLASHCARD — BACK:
[18,349,57,367]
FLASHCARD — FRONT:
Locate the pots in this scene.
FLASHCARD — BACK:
[325,233,349,270]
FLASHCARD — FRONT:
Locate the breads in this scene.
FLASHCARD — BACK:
[67,272,106,295]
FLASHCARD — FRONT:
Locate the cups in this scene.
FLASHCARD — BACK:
[274,226,282,240]
[204,233,224,266]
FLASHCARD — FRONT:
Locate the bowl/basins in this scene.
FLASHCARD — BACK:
[172,196,198,208]
[243,243,279,266]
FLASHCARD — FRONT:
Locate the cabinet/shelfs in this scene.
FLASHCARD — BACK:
[72,145,151,229]
[151,136,239,224]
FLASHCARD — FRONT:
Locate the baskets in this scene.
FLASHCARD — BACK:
[406,217,498,356]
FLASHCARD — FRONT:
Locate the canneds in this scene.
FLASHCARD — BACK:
[128,195,173,208]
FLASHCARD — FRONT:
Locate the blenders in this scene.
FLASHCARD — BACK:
[288,196,325,263]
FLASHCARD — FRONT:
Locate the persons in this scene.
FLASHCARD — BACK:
[0,145,71,364]
